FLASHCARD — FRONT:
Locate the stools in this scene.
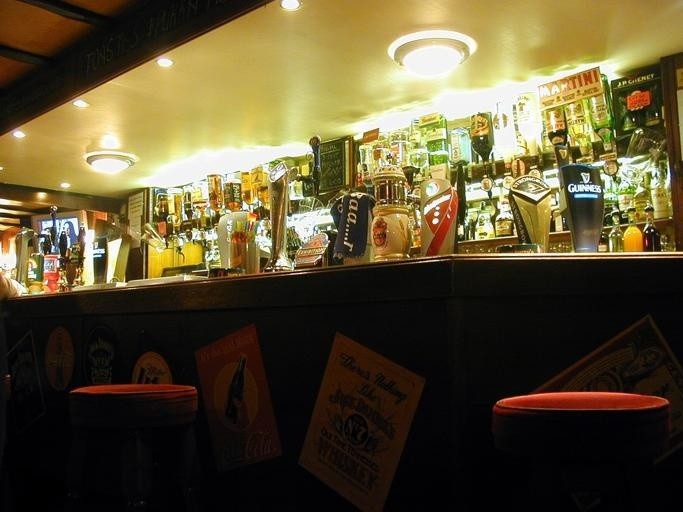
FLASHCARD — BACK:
[67,382,198,511]
[489,389,669,509]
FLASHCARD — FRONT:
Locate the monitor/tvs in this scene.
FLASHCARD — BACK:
[30,209,88,256]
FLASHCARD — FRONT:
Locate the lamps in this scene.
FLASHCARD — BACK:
[83,149,139,176]
[385,30,479,78]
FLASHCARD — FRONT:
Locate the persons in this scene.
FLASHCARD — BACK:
[62,220,78,249]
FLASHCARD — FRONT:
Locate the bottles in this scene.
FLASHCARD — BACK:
[223,355,246,424]
[54,330,66,387]
[88,333,110,383]
[147,75,667,282]
